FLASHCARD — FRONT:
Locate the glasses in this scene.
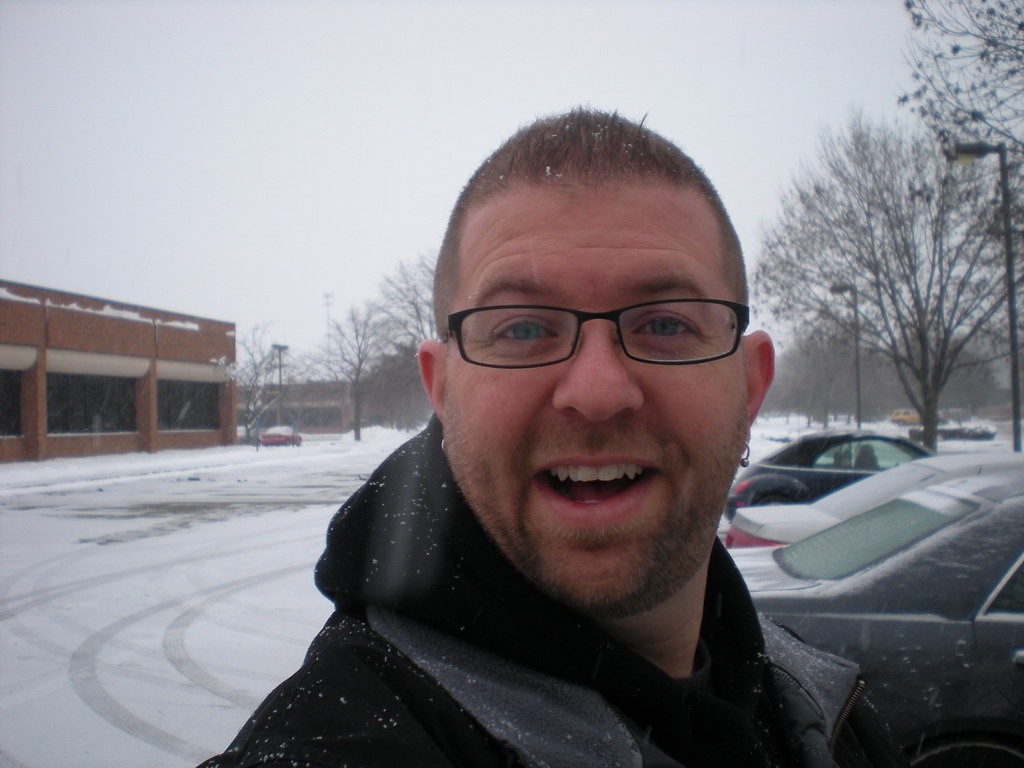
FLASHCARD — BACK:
[440,297,750,371]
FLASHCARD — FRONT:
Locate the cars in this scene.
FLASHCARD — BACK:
[724,430,941,522]
[261,427,302,448]
[722,448,1024,549]
[725,476,1024,768]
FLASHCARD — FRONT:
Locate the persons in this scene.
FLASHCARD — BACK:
[195,113,896,768]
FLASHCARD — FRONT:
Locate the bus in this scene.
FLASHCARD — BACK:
[890,408,945,426]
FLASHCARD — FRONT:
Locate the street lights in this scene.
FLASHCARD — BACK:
[831,283,861,432]
[955,140,1023,455]
[271,344,288,424]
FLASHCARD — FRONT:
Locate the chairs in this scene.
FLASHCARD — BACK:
[855,445,879,468]
